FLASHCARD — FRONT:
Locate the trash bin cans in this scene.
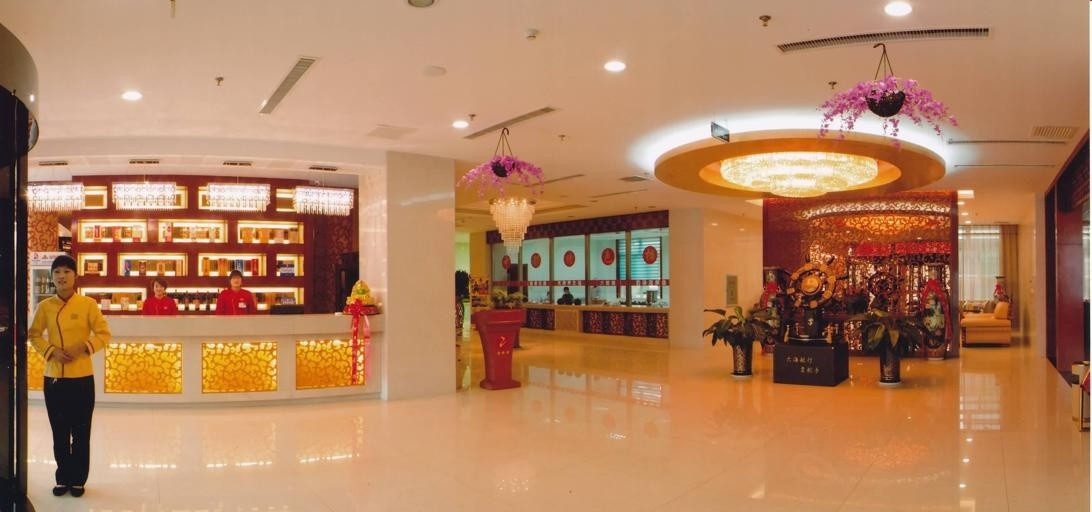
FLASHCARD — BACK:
[475,308,527,390]
[1071,360,1090,422]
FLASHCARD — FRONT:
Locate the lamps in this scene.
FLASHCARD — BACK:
[27,180,86,213]
[111,180,177,213]
[720,150,878,199]
[490,189,536,258]
[293,183,355,219]
[207,178,270,214]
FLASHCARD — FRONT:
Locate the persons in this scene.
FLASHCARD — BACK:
[26,254,112,498]
[574,297,581,306]
[142,277,179,315]
[215,268,258,315]
[562,287,573,304]
[557,297,565,305]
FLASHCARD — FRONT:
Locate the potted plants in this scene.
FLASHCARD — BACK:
[851,309,930,383]
[702,307,773,376]
[494,290,523,349]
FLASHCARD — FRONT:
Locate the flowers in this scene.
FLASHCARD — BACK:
[457,156,545,200]
[816,76,959,152]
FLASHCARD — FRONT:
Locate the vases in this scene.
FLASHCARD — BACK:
[491,162,514,178]
[866,90,904,118]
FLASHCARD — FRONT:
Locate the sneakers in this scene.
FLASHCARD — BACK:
[53,484,69,496]
[70,485,85,497]
